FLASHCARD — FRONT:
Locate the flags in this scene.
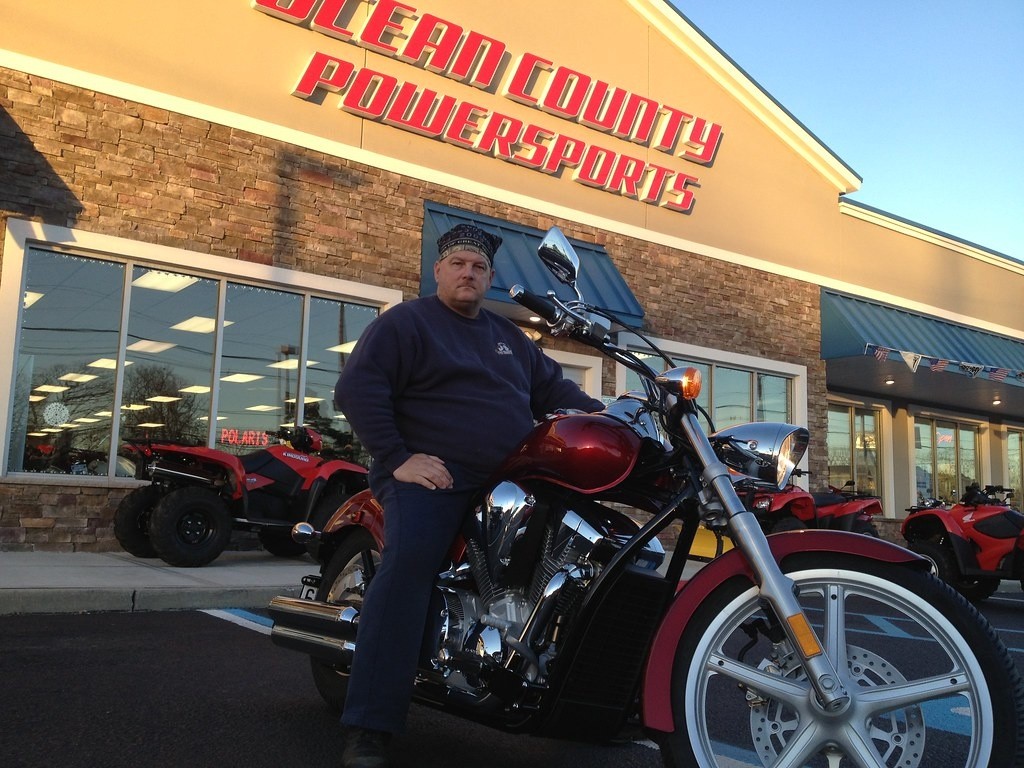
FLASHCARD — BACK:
[989,367,1011,382]
[1015,371,1024,382]
[960,363,983,380]
[930,358,950,372]
[899,351,921,373]
[867,344,889,362]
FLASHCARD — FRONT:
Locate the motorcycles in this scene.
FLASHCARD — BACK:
[111,401,374,568]
[900,480,1024,600]
[727,467,885,541]
[261,223,1024,768]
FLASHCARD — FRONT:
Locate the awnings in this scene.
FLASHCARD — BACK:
[823,291,1024,422]
[420,201,647,336]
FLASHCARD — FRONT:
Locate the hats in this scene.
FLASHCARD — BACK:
[437,224,503,269]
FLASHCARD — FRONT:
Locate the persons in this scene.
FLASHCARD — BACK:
[334,223,607,768]
[970,482,1007,504]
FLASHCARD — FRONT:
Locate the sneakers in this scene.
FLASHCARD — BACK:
[341,726,387,768]
